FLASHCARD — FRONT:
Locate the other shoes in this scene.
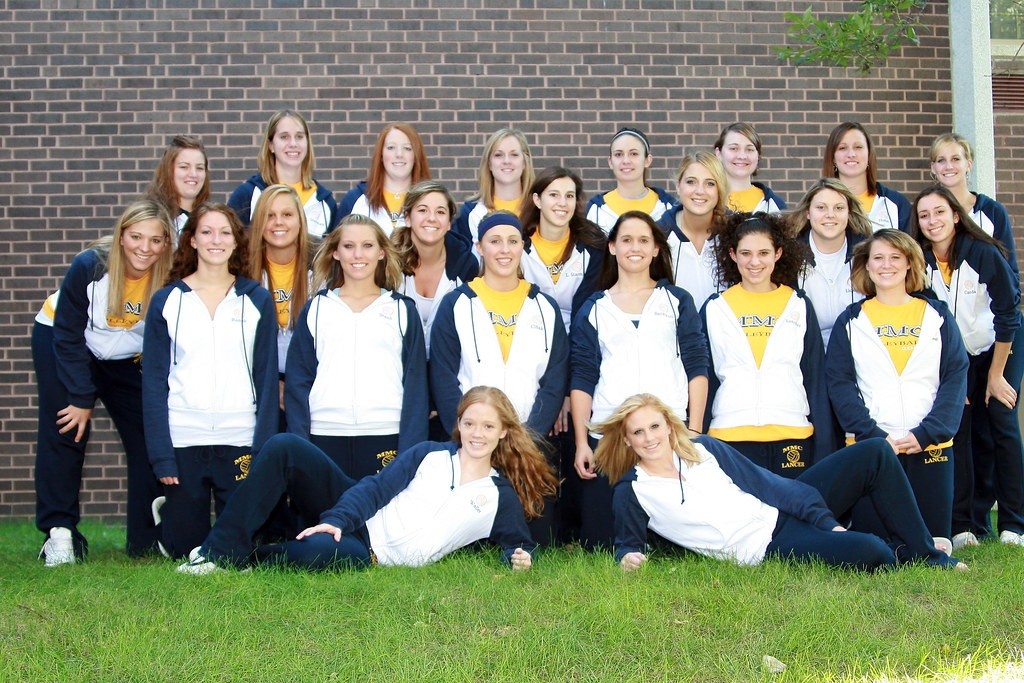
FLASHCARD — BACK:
[932,537,953,557]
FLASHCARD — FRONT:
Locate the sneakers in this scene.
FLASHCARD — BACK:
[173,547,226,575]
[952,531,980,553]
[35,525,77,568]
[1000,531,1020,546]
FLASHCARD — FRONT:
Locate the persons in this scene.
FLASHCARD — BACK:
[142,108,1024,565]
[176,387,560,573]
[32,199,178,568]
[594,393,966,574]
